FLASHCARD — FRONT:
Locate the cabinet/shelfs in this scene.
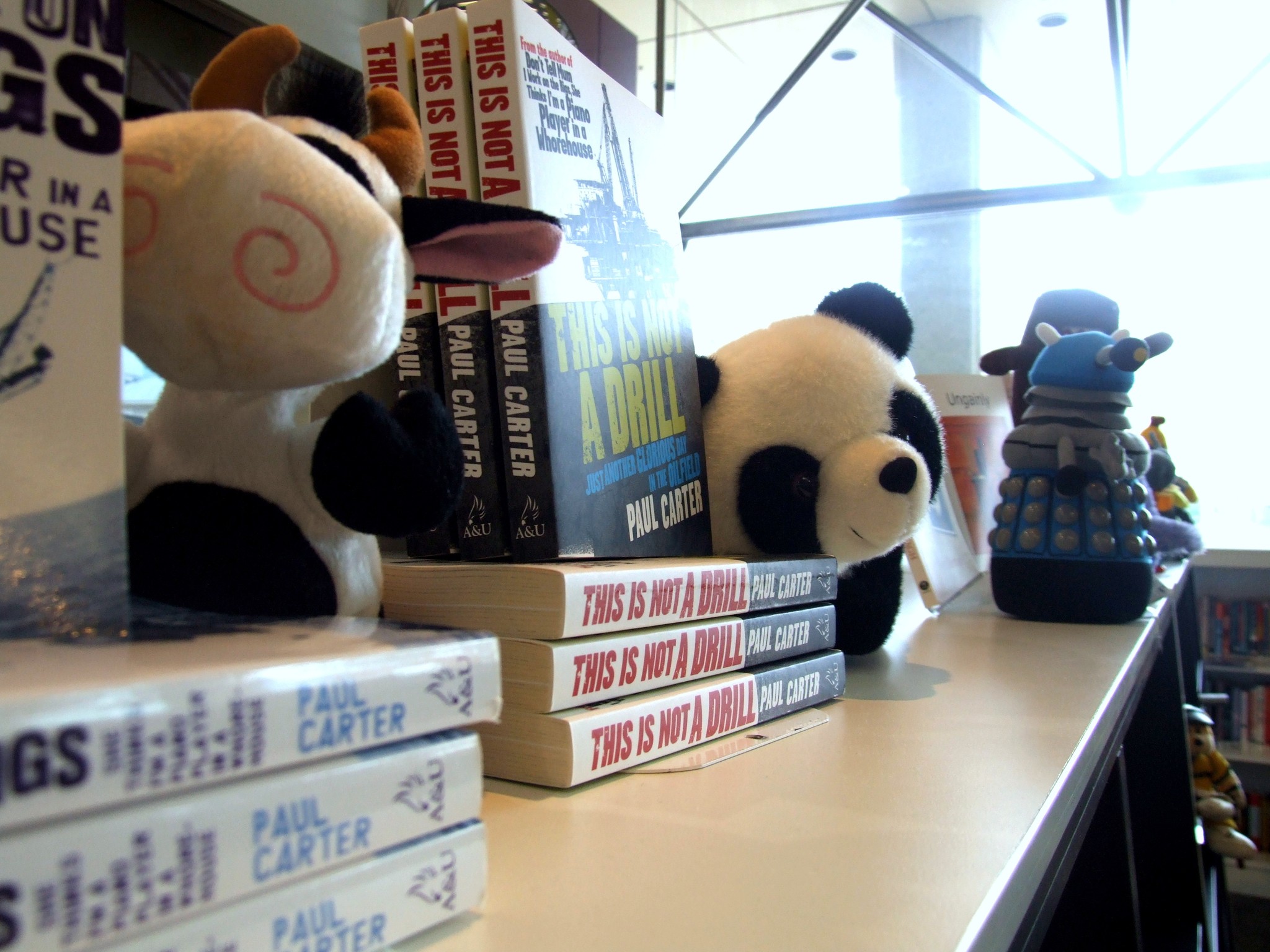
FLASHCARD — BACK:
[1192,545,1270,899]
[406,550,1233,952]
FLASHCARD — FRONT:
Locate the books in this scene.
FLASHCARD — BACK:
[1196,590,1270,854]
[0,0,848,952]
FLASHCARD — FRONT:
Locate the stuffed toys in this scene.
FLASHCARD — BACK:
[695,282,945,655]
[1183,704,1258,860]
